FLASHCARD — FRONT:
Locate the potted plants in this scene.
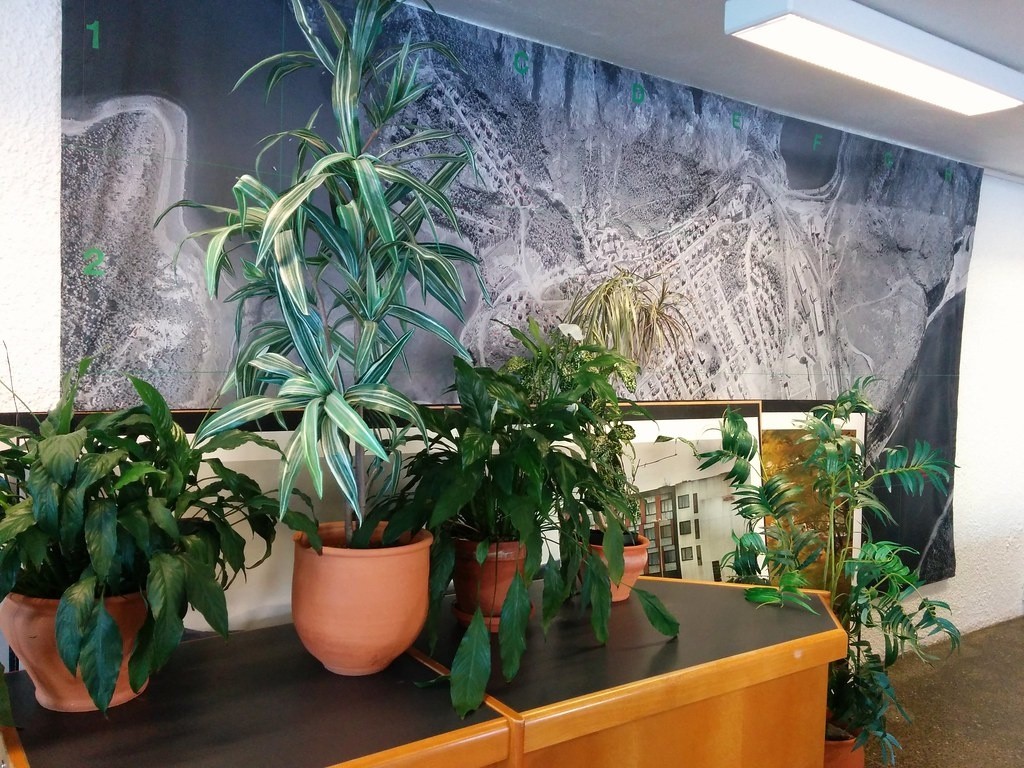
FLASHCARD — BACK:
[693,373,960,768]
[349,263,697,721]
[0,351,324,722]
[152,0,491,677]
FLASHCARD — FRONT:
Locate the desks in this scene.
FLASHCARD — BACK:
[0,575,849,768]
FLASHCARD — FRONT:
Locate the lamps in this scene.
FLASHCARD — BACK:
[724,0,1024,115]
